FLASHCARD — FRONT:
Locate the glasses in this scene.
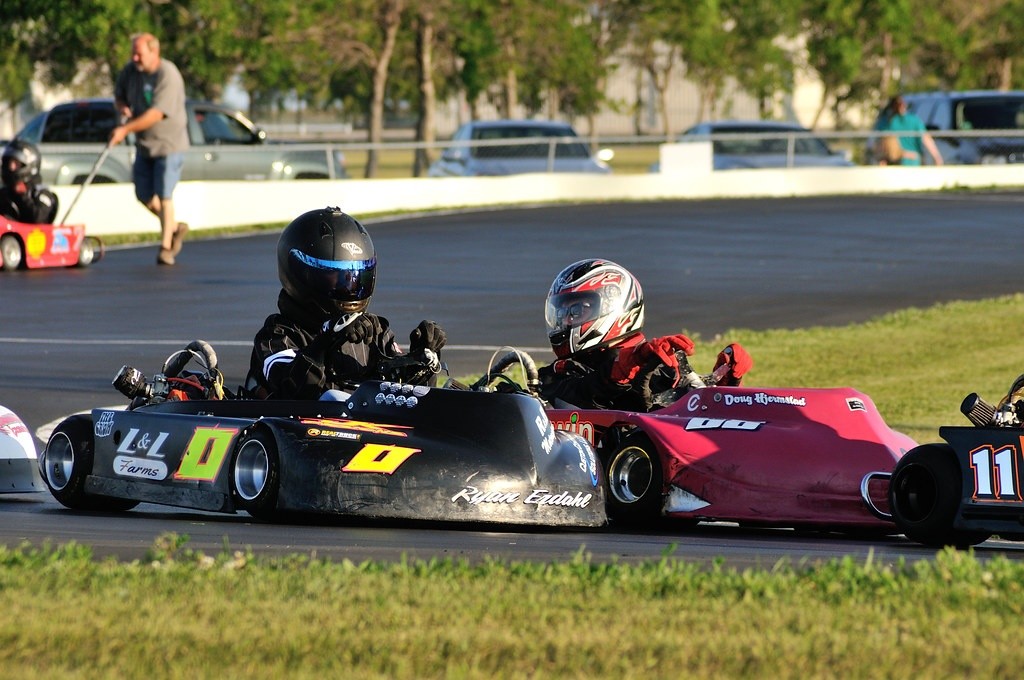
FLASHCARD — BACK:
[557,302,593,319]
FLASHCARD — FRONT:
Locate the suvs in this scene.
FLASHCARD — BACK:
[1,99,350,180]
[868,89,1024,165]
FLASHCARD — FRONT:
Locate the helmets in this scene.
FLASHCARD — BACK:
[544,258,645,358]
[1,141,41,183]
[277,206,377,314]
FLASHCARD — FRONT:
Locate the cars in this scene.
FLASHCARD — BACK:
[650,120,856,168]
[426,121,615,178]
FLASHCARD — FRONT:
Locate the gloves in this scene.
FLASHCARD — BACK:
[619,334,695,379]
[317,312,383,344]
[713,343,753,379]
[410,320,447,353]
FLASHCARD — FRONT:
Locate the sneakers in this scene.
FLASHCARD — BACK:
[157,223,188,265]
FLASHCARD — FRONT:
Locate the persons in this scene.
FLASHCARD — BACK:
[872,94,943,166]
[253,207,447,401]
[537,258,752,413]
[110,33,189,265]
[0,140,54,224]
